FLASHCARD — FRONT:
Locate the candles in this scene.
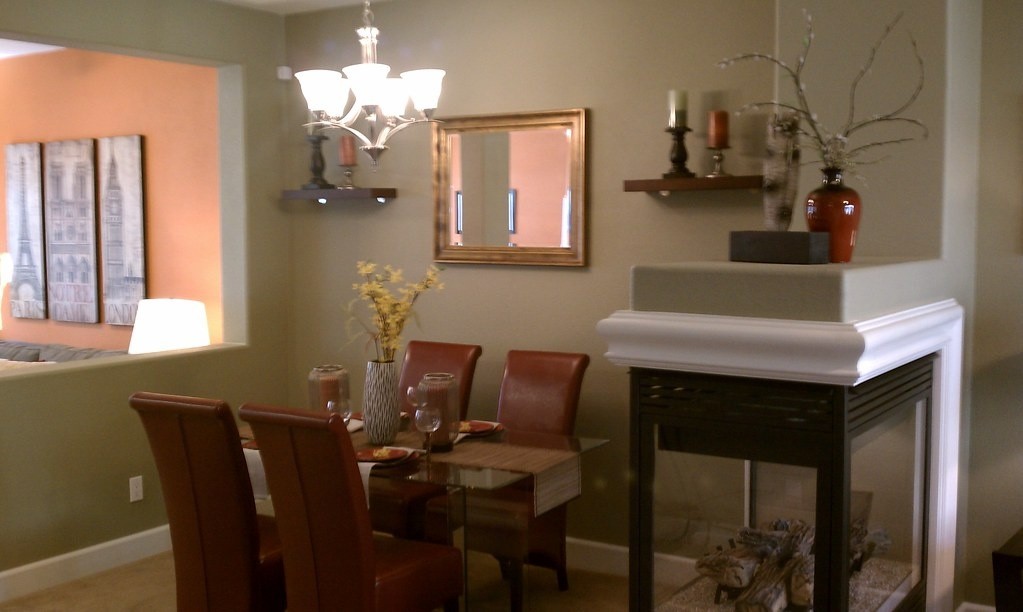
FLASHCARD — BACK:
[707,111,728,147]
[338,135,356,166]
[668,87,688,128]
[307,105,322,136]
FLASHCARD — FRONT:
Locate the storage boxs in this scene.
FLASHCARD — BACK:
[597,296,965,612]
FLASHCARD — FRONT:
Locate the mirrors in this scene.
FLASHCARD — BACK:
[429,107,590,265]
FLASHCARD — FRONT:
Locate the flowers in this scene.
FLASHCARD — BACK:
[342,259,446,361]
[716,7,931,168]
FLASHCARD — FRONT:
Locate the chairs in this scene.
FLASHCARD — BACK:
[130,341,592,612]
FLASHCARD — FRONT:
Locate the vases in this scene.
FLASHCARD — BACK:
[364,360,401,446]
[802,169,863,262]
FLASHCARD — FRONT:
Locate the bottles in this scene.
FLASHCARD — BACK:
[418,373,461,449]
[307,364,351,411]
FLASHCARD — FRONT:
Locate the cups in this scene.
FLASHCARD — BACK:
[327,399,351,423]
[407,385,429,410]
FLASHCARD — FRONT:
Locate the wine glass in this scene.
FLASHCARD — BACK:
[414,407,440,462]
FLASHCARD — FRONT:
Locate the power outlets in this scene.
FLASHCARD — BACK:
[129,475,144,502]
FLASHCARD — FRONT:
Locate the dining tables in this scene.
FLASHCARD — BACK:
[242,426,613,612]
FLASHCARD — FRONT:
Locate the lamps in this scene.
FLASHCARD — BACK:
[127,299,210,355]
[294,0,448,172]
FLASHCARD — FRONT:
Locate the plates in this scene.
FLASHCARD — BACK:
[454,420,504,437]
[356,448,420,470]
